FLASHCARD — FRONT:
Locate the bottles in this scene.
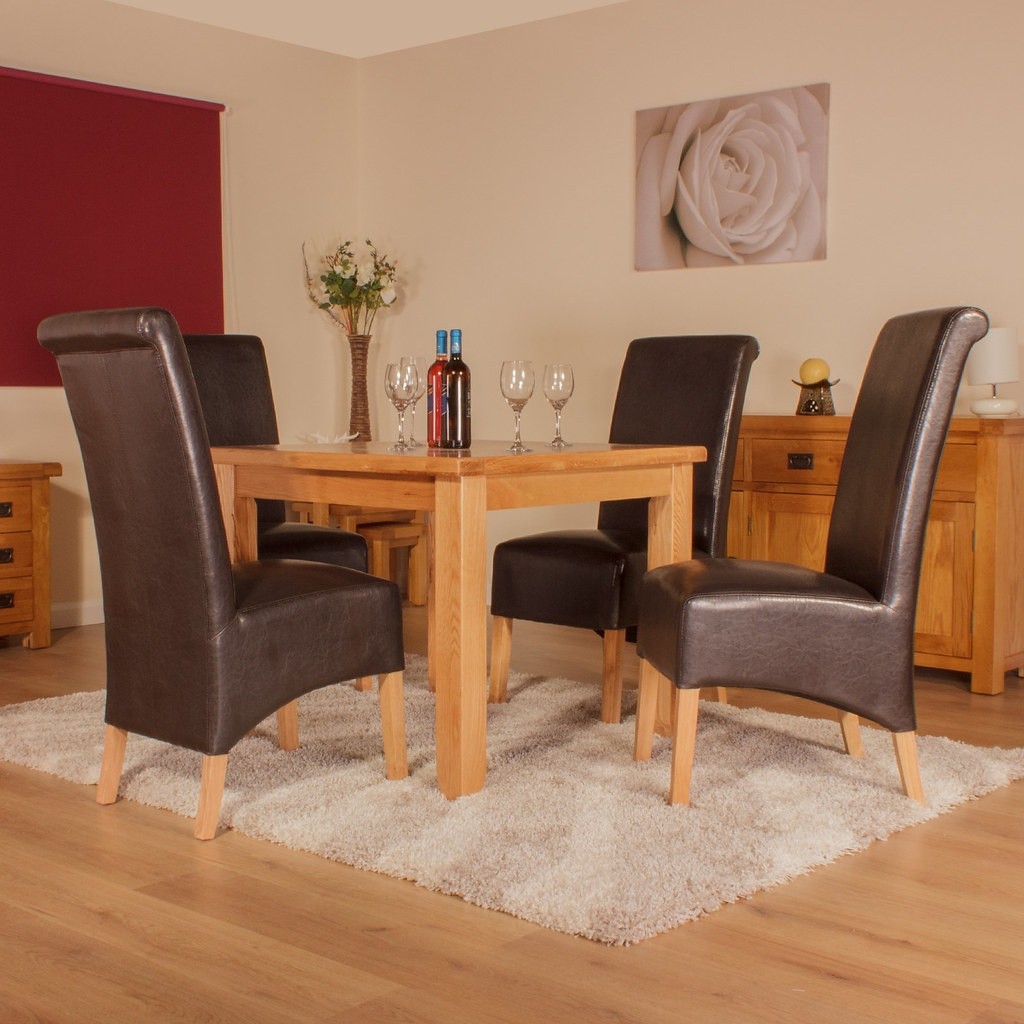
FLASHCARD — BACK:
[425,329,449,447]
[439,328,472,448]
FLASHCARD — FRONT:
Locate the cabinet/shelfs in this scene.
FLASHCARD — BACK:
[716,413,1024,700]
[0,461,64,655]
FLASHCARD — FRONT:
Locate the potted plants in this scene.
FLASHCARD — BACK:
[343,328,376,443]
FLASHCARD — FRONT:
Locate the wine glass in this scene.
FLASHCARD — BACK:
[542,361,576,447]
[501,360,536,452]
[398,356,429,447]
[383,363,418,451]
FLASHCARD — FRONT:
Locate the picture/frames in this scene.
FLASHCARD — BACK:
[628,81,835,269]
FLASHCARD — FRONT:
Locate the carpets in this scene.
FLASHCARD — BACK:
[0,627,1024,950]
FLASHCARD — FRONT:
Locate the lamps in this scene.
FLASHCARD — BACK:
[961,326,1021,421]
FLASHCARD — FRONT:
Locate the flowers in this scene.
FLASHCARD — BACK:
[300,237,400,333]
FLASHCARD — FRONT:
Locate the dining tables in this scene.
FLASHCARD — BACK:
[201,427,716,803]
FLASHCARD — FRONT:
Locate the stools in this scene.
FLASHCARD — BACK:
[357,521,430,609]
[293,499,418,532]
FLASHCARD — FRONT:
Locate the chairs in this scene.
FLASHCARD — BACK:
[487,332,761,728]
[177,328,383,696]
[629,305,995,811]
[34,304,418,841]
